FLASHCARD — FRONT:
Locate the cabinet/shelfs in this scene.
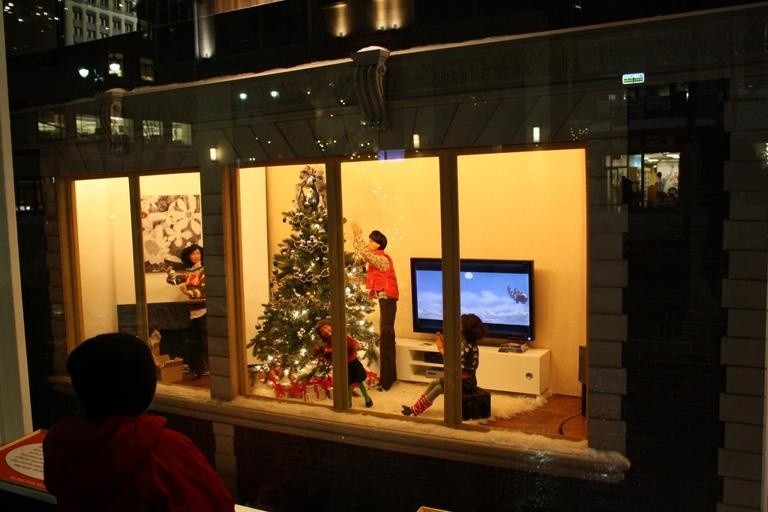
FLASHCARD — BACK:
[370,337,551,396]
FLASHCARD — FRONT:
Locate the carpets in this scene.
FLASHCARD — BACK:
[247,380,552,426]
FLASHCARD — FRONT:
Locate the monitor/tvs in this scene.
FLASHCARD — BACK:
[411,257,535,341]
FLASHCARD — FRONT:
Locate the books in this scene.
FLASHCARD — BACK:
[498,342,528,353]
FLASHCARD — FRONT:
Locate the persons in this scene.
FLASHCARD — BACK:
[653,166,674,195]
[315,320,373,409]
[402,314,486,417]
[351,221,399,392]
[166,244,207,381]
[42,332,236,512]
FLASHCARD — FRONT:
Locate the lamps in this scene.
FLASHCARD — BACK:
[532,125,540,148]
[207,143,218,163]
[412,133,421,153]
[77,64,92,78]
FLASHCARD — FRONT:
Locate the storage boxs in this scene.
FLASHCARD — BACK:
[155,354,184,383]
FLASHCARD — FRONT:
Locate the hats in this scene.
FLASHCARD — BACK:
[68,331,156,417]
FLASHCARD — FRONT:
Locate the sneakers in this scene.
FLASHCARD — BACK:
[402,409,417,416]
[402,405,411,409]
[366,397,373,407]
